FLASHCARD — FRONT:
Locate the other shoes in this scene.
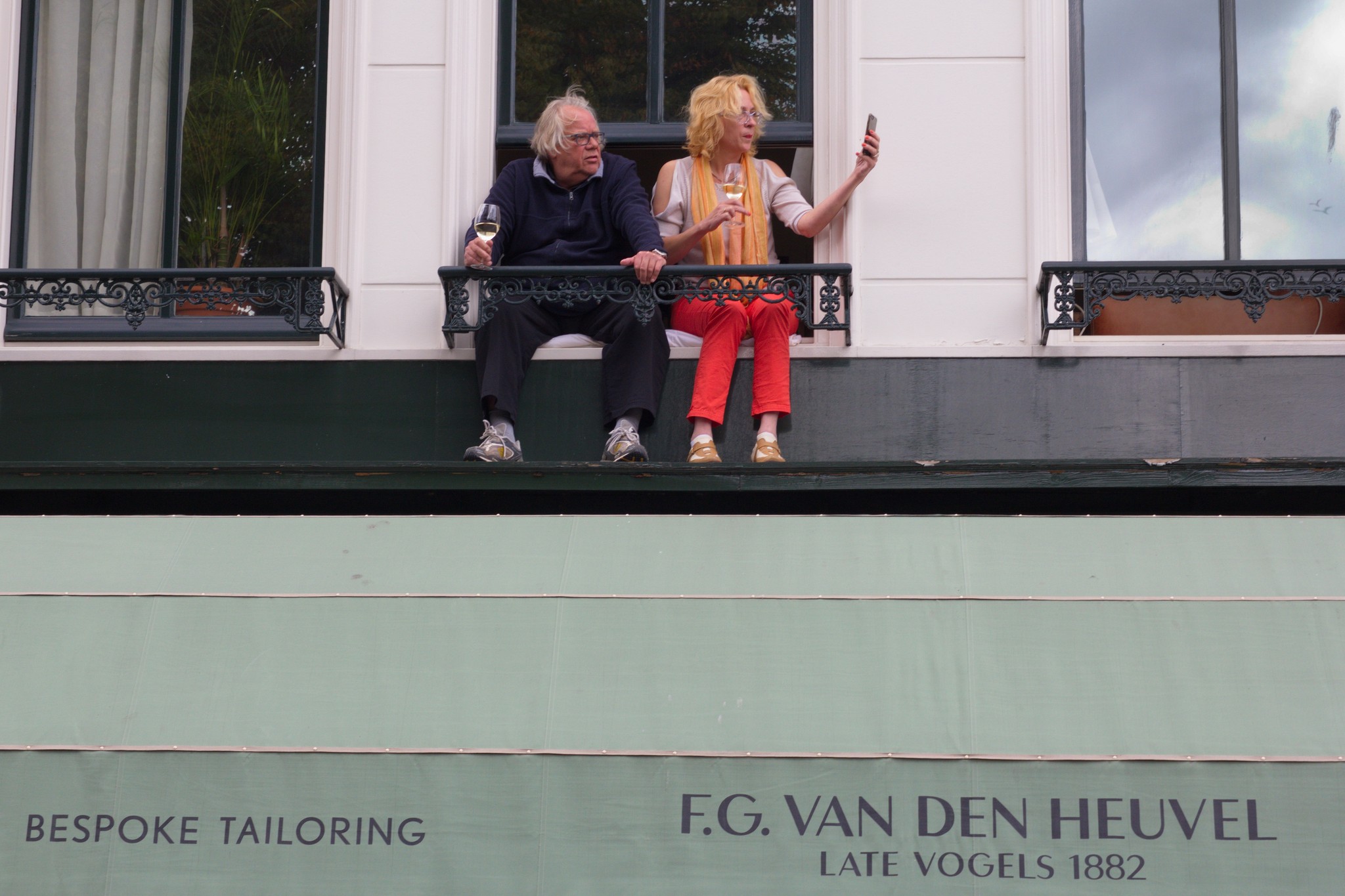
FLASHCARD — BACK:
[686,439,722,463]
[750,438,786,463]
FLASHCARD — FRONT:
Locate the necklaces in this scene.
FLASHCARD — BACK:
[711,172,738,184]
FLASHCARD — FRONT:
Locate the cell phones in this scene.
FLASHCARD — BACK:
[862,114,877,155]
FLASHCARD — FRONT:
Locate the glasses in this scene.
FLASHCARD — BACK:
[717,108,763,126]
[563,131,606,147]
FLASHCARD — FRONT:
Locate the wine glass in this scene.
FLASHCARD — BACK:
[470,203,501,270]
[722,163,747,228]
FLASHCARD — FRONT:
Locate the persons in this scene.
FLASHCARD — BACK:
[649,73,880,465]
[461,84,673,461]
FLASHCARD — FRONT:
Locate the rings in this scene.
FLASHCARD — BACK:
[724,207,727,213]
[873,150,880,157]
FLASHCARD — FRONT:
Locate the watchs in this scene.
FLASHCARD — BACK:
[650,248,667,260]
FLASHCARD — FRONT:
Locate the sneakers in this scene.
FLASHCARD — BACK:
[463,418,524,462]
[600,419,649,462]
